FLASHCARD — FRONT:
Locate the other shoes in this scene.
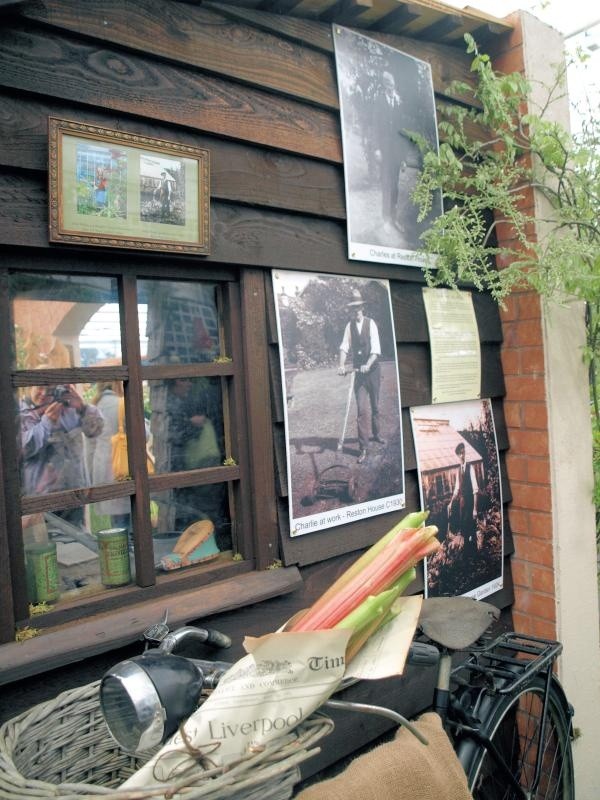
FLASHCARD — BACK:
[357,449,367,462]
[374,436,385,444]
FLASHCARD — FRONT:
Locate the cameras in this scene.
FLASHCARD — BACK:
[53,385,72,409]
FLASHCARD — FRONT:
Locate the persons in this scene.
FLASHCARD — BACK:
[446,443,480,565]
[369,71,408,234]
[19,363,232,582]
[338,289,387,464]
[153,172,172,218]
[91,168,109,212]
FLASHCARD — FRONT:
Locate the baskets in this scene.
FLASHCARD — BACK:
[1,679,334,799]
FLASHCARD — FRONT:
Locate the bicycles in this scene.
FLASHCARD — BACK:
[97,595,577,800]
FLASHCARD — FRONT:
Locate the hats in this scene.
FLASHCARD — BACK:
[344,289,367,306]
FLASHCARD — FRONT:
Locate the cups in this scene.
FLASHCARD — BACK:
[23,541,60,605]
[95,527,132,588]
